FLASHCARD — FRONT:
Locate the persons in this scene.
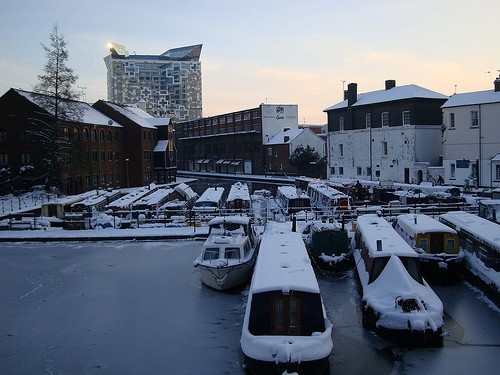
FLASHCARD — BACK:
[355,179,362,190]
[437,176,444,185]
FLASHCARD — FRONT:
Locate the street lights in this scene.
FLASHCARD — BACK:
[125,158,130,188]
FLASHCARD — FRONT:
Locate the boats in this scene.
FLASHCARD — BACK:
[193,216,261,290]
[40,178,467,219]
[242,212,333,364]
[352,215,445,342]
[439,212,500,294]
[393,212,465,288]
[299,219,354,280]
